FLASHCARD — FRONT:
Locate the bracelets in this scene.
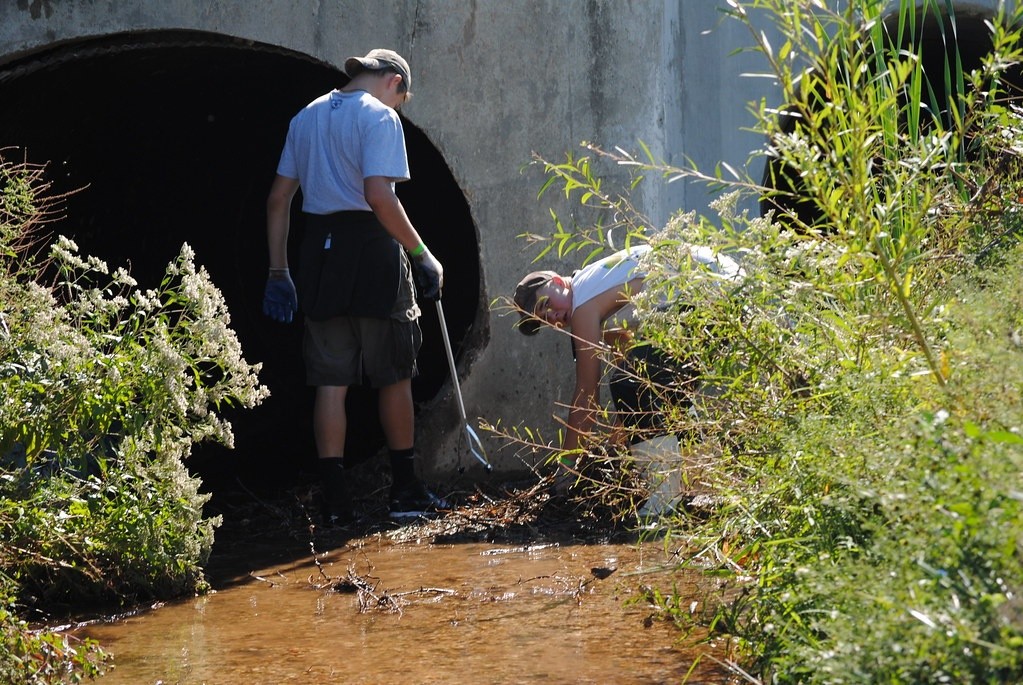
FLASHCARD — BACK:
[409,242,425,258]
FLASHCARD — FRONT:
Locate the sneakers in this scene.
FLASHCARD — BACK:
[389,493,454,518]
[321,507,367,527]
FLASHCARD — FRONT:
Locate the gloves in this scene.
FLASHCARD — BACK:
[412,247,444,300]
[261,268,298,324]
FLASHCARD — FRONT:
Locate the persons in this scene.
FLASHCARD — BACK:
[262,49,451,518]
[513,241,748,516]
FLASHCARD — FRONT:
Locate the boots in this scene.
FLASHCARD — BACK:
[619,432,687,519]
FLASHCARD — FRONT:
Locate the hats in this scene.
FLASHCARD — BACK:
[344,48,412,91]
[515,270,556,335]
